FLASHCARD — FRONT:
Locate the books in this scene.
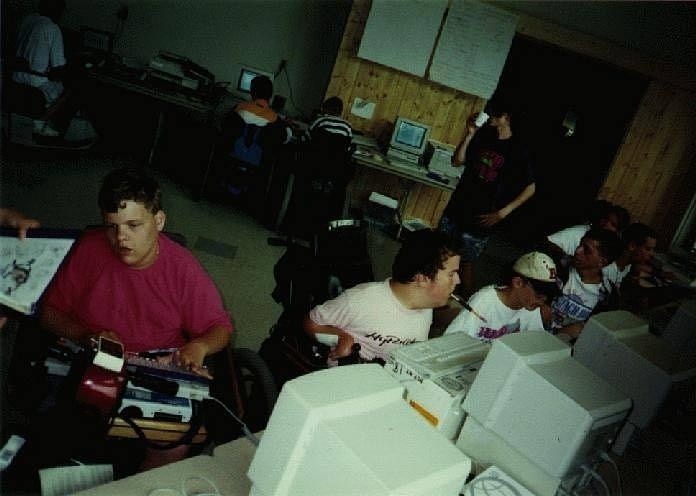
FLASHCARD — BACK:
[0,235,77,318]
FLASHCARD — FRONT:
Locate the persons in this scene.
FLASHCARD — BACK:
[540,225,627,344]
[545,204,631,260]
[187,73,293,204]
[298,94,355,167]
[300,226,464,373]
[437,89,537,288]
[444,251,564,340]
[0,159,235,374]
[1,0,66,113]
[603,222,680,294]
[0,202,45,331]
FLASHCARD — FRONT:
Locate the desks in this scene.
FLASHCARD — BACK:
[352,144,458,240]
[62,60,216,167]
[63,429,267,495]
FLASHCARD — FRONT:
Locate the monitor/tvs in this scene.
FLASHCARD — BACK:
[235,66,274,98]
[571,309,696,459]
[386,115,432,164]
[661,299,696,352]
[245,362,472,496]
[456,329,633,496]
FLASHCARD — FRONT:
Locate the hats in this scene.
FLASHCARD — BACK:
[512,251,563,297]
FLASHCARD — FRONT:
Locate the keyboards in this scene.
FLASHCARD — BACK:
[397,331,492,378]
[387,157,429,174]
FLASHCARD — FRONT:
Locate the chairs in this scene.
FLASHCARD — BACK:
[1,45,98,160]
[211,110,357,241]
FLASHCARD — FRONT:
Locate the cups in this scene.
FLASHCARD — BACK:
[474,111,490,128]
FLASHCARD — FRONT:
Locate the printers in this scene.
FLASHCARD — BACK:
[423,140,465,178]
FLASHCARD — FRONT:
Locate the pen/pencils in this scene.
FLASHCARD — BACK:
[450,293,487,323]
